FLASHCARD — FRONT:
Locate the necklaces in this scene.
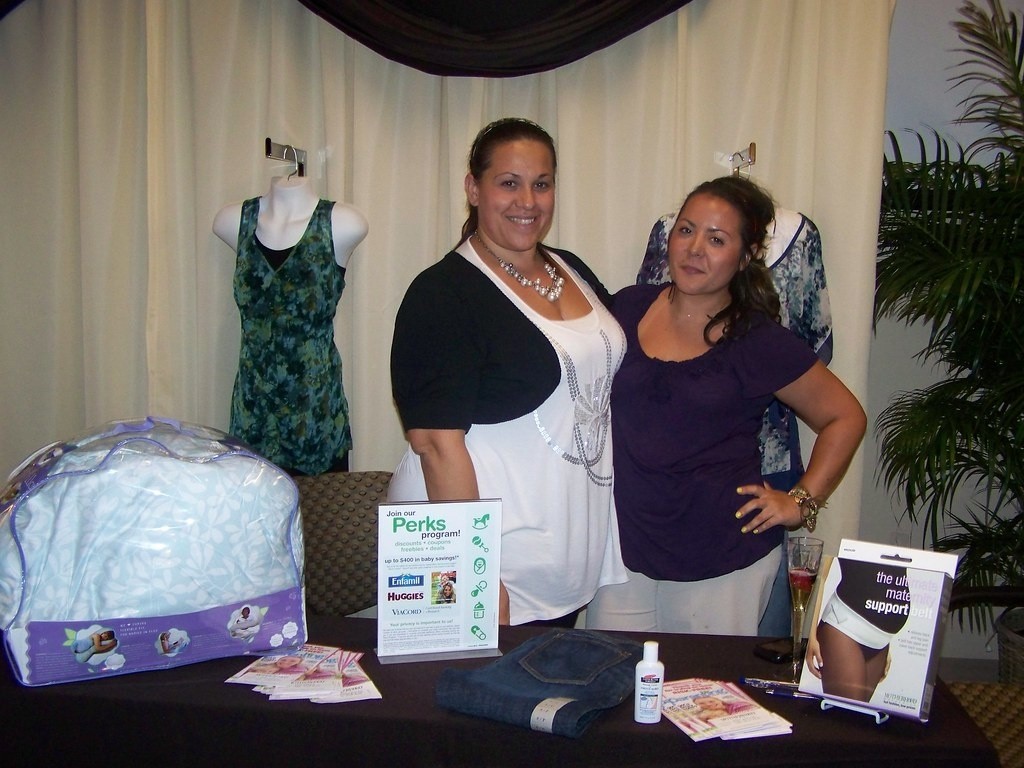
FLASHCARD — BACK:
[475,230,564,300]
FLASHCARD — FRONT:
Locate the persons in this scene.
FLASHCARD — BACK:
[436,571,457,604]
[637,206,834,496]
[73,631,118,652]
[162,632,184,652]
[806,559,911,704]
[692,695,753,720]
[586,176,868,638]
[389,118,628,627]
[231,607,258,636]
[253,655,308,675]
[211,174,369,477]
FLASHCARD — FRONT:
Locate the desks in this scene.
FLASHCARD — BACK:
[0,619,1001,768]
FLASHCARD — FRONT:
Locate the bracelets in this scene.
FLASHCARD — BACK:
[788,487,818,531]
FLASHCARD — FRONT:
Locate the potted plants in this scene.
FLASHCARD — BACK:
[869,0,1024,688]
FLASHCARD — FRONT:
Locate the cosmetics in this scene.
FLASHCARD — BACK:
[636,640,665,724]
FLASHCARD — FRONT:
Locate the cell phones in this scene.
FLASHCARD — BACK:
[754,637,808,664]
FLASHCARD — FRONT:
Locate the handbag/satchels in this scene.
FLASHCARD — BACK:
[0,415,304,685]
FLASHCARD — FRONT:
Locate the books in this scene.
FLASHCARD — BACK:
[661,678,794,742]
[225,643,384,703]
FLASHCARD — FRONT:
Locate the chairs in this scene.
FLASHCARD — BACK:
[289,471,393,618]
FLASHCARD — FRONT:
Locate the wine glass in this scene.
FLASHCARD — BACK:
[772,537,824,683]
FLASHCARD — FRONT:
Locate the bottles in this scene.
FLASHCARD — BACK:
[634,641,664,723]
